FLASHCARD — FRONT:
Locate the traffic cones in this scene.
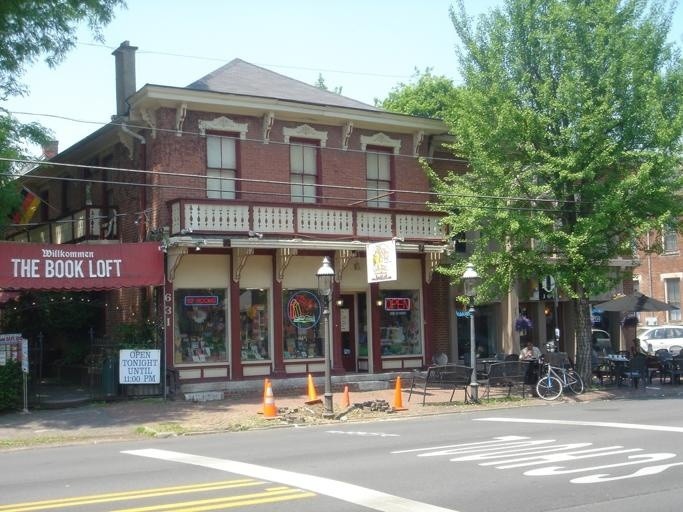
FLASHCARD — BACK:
[336,384,355,416]
[304,373,323,405]
[388,376,408,412]
[256,377,279,414]
[259,381,282,420]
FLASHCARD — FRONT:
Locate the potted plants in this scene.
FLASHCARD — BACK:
[455,294,471,313]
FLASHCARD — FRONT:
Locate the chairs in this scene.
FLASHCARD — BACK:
[469,344,682,407]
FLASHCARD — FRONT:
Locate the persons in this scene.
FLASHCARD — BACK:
[518,341,545,384]
[632,337,649,358]
[6,187,42,231]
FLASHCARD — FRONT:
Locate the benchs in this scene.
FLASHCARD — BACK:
[405,362,473,408]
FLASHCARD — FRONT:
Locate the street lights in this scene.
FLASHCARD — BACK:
[315,255,336,420]
[460,262,483,404]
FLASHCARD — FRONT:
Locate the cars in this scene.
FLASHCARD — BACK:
[636,327,653,339]
[636,325,683,353]
[248,327,286,352]
[358,318,407,354]
[546,328,612,353]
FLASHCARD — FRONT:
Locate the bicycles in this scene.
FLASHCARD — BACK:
[535,357,584,400]
